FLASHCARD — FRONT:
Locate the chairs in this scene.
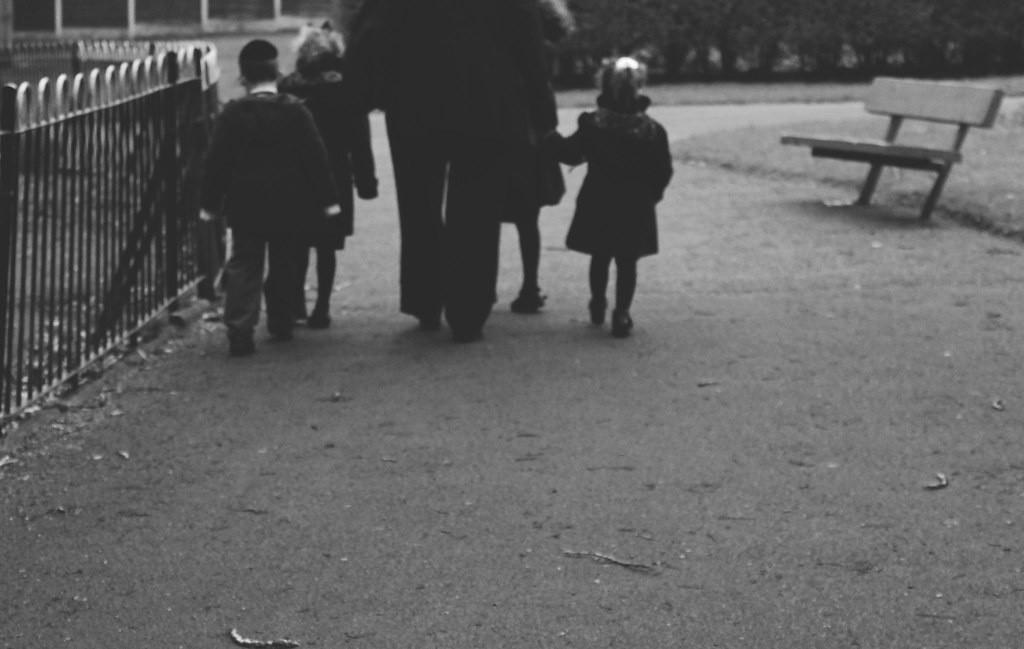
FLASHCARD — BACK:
[781,75,1004,224]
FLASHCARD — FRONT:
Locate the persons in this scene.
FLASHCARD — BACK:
[342,0,559,344]
[536,55,675,338]
[497,0,578,314]
[197,39,342,358]
[274,20,378,330]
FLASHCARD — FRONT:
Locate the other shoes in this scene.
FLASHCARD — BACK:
[229,330,254,357]
[510,285,546,311]
[454,323,486,344]
[590,294,608,326]
[418,308,445,330]
[308,303,333,326]
[614,307,633,337]
[270,318,296,339]
